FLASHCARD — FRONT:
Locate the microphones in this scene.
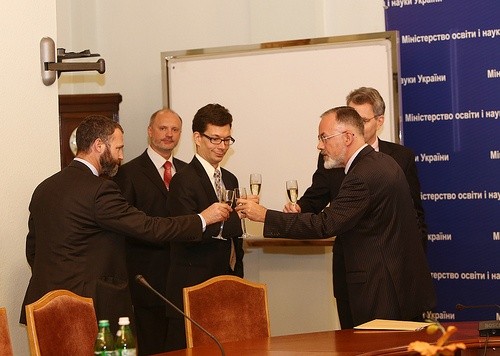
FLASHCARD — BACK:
[136,272,225,356]
[456,304,500,336]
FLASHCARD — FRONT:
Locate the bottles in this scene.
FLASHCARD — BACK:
[93,320,122,356]
[115,317,137,356]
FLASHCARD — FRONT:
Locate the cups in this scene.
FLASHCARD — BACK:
[249,173,263,196]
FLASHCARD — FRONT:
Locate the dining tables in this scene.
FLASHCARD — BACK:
[148,321,500,356]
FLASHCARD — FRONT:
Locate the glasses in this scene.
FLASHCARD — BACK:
[362,113,383,124]
[318,131,354,144]
[199,131,236,145]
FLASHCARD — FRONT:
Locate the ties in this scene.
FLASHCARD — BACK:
[163,160,172,191]
[214,169,222,201]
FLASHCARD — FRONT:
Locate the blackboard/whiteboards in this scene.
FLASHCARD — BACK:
[159,27,404,245]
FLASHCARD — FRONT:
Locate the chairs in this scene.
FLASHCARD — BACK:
[183,274,271,348]
[25,289,98,356]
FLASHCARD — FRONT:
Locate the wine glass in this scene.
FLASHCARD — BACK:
[234,187,253,239]
[286,180,298,208]
[212,190,234,240]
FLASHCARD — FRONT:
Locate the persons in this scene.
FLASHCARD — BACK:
[234,105,440,330]
[113,107,189,356]
[168,102,244,349]
[18,113,233,335]
[282,86,429,257]
